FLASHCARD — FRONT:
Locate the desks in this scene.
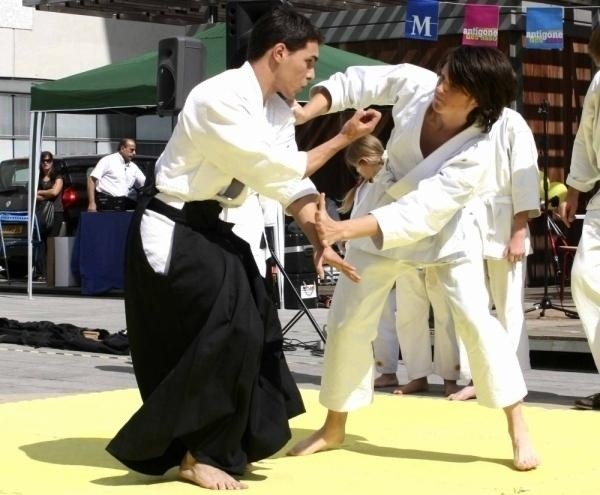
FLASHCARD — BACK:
[78,207,135,298]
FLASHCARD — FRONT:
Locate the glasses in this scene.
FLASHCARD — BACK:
[41,158,52,163]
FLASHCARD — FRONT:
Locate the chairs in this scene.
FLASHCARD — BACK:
[0,209,48,286]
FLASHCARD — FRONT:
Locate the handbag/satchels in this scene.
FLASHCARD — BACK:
[36,200,55,231]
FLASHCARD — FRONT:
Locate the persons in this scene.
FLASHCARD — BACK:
[334,135,399,388]
[558,26,600,412]
[444,107,541,405]
[87,137,147,213]
[218,186,278,283]
[33,150,69,283]
[393,265,464,397]
[104,4,385,495]
[282,43,541,473]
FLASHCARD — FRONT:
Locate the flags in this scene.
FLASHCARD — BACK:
[405,2,439,43]
[524,7,564,49]
[462,5,499,47]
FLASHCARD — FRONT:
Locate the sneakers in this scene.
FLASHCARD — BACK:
[575,393,599,410]
[31,275,47,284]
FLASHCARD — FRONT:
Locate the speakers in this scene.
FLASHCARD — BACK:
[157,37,205,115]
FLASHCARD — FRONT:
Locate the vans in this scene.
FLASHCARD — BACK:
[1,154,164,279]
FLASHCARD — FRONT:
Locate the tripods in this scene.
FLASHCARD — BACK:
[524,98,580,320]
